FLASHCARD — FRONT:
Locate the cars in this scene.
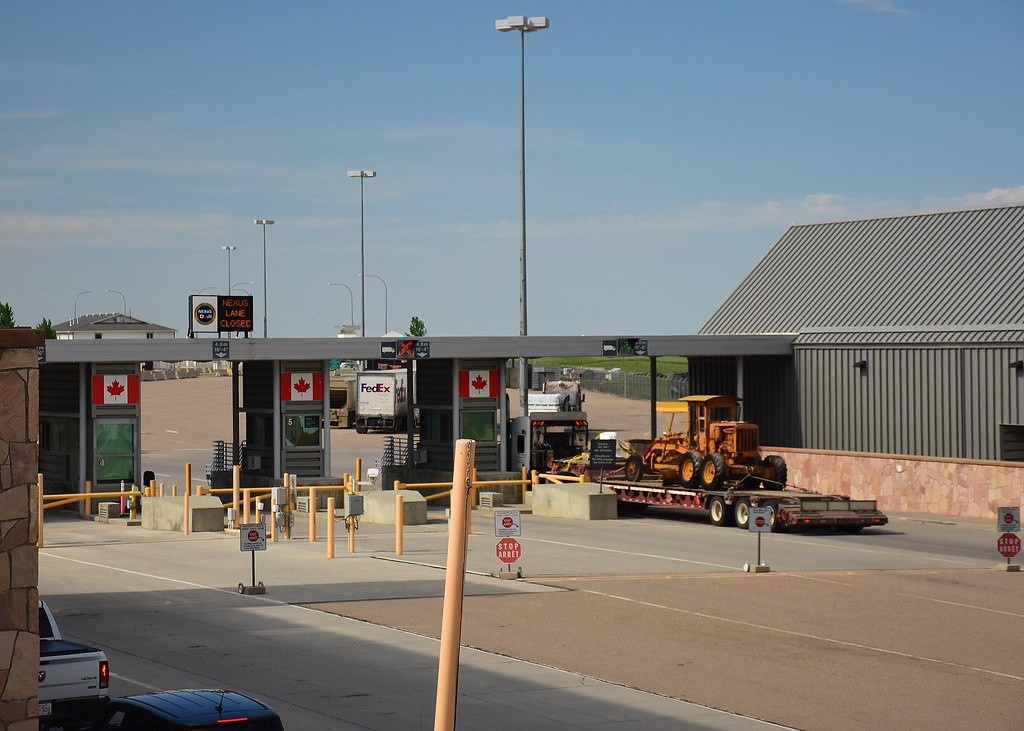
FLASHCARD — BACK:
[62,687,286,731]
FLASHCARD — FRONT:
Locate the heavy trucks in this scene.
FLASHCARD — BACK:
[506,411,889,532]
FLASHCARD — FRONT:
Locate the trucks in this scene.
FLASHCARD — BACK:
[355,368,416,434]
[521,378,587,417]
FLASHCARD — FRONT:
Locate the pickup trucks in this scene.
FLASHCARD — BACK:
[35,598,115,726]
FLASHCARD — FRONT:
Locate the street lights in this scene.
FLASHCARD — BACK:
[222,245,236,338]
[494,14,550,422]
[253,220,275,338]
[73,290,97,322]
[347,168,377,336]
[105,289,126,316]
[358,273,388,334]
[327,282,354,327]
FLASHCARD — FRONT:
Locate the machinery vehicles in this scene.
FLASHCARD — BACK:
[624,395,787,491]
